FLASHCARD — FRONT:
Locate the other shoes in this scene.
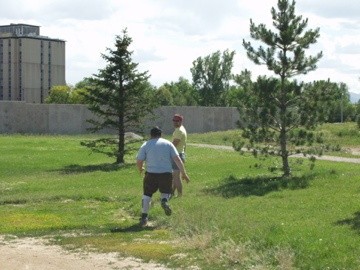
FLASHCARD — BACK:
[140,218,148,224]
[161,201,171,216]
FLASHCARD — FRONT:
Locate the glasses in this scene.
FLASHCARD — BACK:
[172,118,180,123]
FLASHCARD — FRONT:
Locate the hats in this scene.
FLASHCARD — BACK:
[151,127,161,134]
[172,115,183,121]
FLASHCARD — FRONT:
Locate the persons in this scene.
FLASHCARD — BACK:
[136,126,191,226]
[170,114,187,199]
